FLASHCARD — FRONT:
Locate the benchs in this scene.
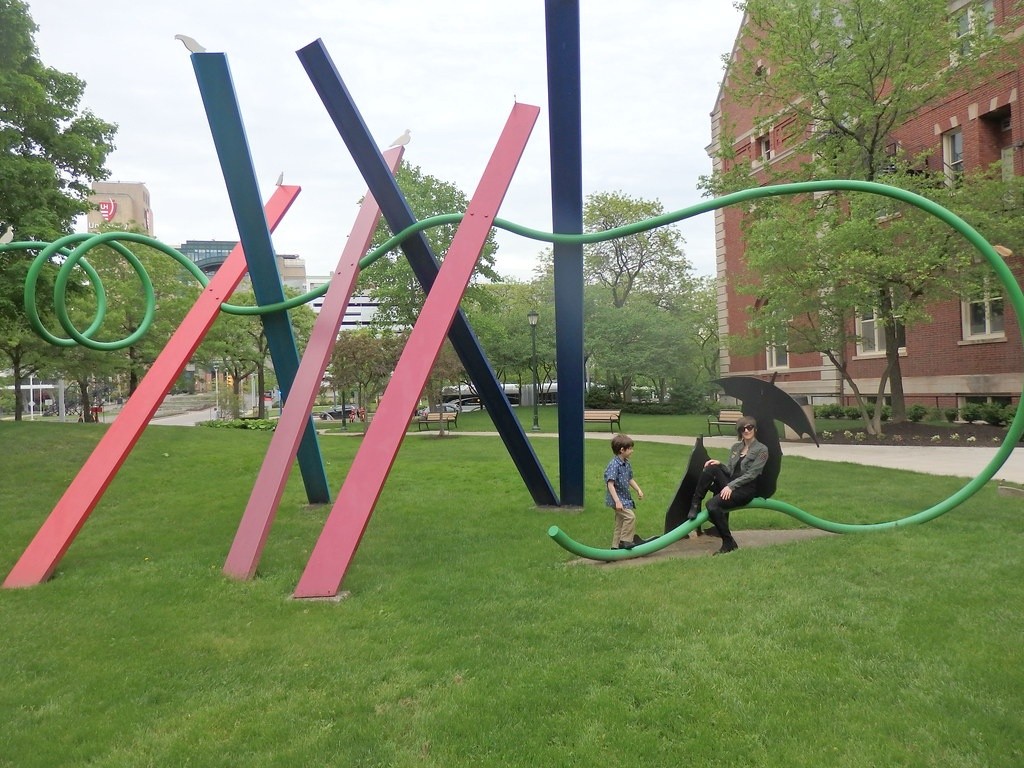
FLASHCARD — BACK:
[418,411,458,432]
[583,408,622,434]
[707,409,744,438]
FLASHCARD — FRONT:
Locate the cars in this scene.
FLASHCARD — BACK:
[321,405,357,419]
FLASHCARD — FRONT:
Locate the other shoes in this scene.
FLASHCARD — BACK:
[619,542,634,549]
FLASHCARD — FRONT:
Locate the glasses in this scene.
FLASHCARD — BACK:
[739,424,754,432]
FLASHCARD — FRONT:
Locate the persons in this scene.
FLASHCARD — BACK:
[603,434,643,550]
[687,416,769,556]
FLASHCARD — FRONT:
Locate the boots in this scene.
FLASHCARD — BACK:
[710,508,738,556]
[688,472,714,520]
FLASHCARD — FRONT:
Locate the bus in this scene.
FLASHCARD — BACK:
[443,383,600,411]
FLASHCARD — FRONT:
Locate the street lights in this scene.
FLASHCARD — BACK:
[213,364,219,407]
[527,308,541,431]
